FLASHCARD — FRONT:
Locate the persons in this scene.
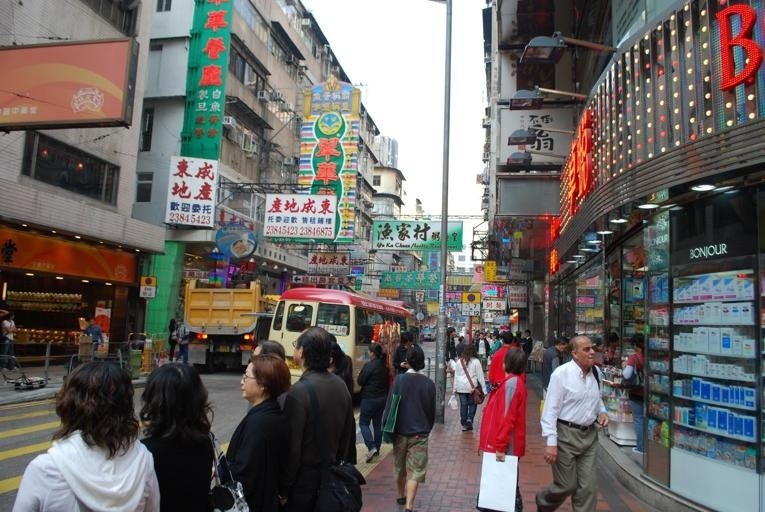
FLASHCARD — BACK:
[0,313,645,511]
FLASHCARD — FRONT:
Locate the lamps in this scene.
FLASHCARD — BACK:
[520,31,616,62]
[507,149,566,170]
[635,199,660,209]
[508,126,574,145]
[563,207,630,263]
[249,257,297,275]
[688,181,718,192]
[509,86,586,113]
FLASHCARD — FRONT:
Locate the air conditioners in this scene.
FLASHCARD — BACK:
[359,193,374,209]
[223,116,236,130]
[283,156,295,166]
[257,88,294,112]
[301,17,341,75]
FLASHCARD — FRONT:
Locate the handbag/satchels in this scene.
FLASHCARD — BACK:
[471,385,484,404]
[209,480,251,512]
[629,384,644,400]
[383,394,402,443]
[314,460,365,512]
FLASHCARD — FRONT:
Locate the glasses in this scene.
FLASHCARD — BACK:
[583,346,598,352]
[242,373,256,382]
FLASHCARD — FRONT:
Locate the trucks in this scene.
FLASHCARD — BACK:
[178,277,283,373]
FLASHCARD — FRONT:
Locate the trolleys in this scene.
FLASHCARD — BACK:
[0,354,48,389]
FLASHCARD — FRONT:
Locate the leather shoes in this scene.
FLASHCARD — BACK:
[397,496,406,504]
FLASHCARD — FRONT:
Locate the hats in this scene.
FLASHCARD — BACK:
[407,345,424,370]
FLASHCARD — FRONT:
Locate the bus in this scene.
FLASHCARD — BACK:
[267,288,418,395]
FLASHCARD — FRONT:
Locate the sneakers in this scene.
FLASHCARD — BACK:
[633,447,643,454]
[461,417,473,431]
[366,448,377,462]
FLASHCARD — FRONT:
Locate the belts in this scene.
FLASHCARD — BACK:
[557,418,594,430]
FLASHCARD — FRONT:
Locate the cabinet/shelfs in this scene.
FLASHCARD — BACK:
[601,363,637,446]
[647,267,671,448]
[575,266,603,336]
[8,289,87,343]
[670,255,765,473]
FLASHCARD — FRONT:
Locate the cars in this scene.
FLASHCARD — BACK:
[422,328,436,341]
